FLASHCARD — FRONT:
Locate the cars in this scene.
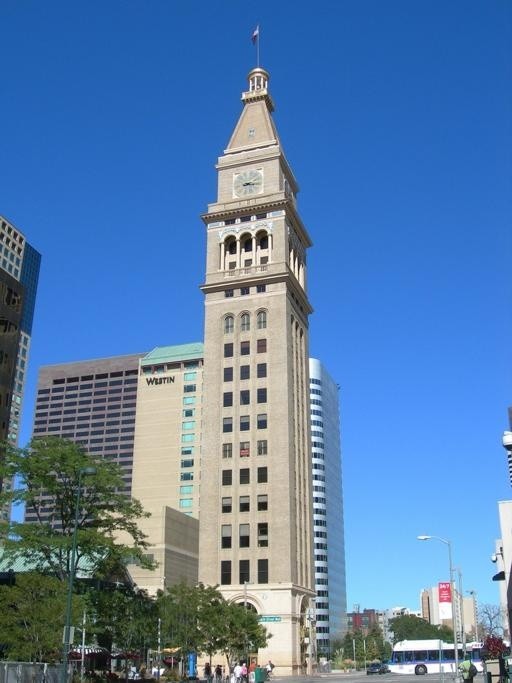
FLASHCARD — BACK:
[367,657,391,674]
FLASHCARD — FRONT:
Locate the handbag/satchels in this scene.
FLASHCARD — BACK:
[468,664,477,678]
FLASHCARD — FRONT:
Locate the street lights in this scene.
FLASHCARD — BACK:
[60,465,98,682]
[452,566,468,661]
[242,580,255,660]
[414,534,462,682]
[312,597,317,663]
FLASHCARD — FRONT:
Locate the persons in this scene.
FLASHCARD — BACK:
[202,659,275,683]
[457,652,473,682]
[302,659,307,679]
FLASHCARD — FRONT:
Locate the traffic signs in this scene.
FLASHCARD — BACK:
[253,614,282,622]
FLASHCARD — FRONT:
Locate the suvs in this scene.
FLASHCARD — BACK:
[128,658,168,678]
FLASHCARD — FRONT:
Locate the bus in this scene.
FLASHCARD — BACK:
[387,634,512,676]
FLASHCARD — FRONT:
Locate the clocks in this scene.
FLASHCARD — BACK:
[231,167,265,201]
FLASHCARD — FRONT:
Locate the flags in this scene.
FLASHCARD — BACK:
[250,24,259,45]
